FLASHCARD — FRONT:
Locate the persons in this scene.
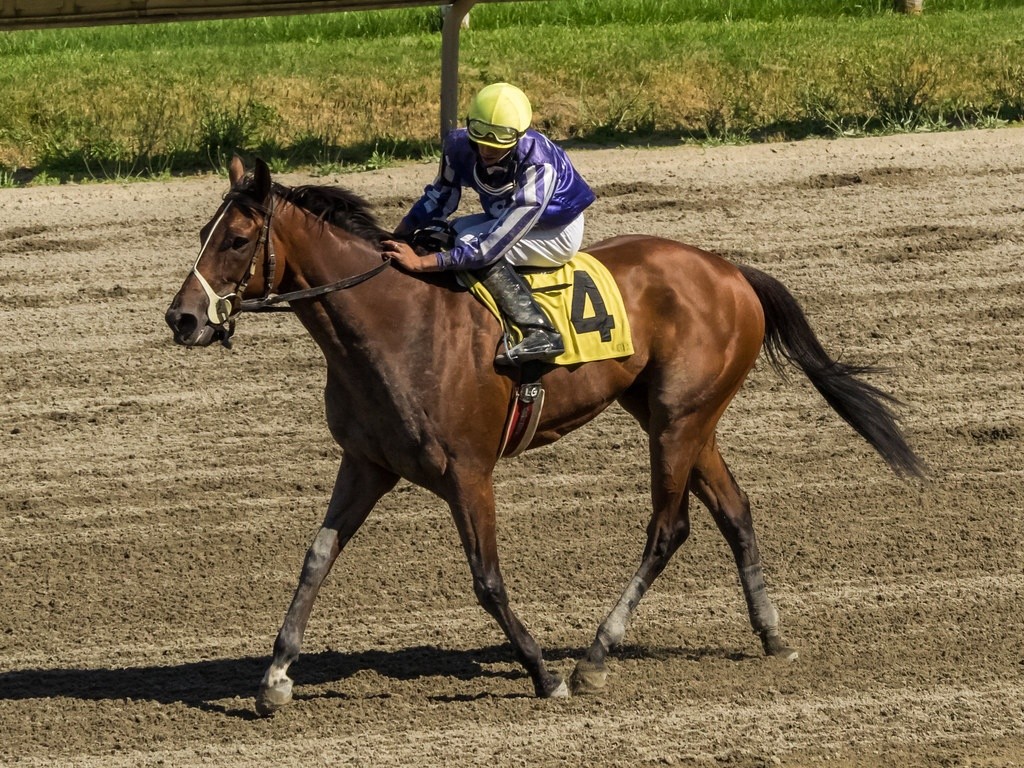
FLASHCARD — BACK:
[378,82,584,366]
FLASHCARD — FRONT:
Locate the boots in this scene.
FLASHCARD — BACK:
[483,264,565,365]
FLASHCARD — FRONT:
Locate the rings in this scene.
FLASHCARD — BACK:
[393,244,398,250]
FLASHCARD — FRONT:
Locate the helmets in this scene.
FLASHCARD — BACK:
[468,83,532,149]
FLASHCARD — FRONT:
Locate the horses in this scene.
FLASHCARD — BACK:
[164,147,938,714]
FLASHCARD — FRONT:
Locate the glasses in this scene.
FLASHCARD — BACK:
[467,118,518,144]
